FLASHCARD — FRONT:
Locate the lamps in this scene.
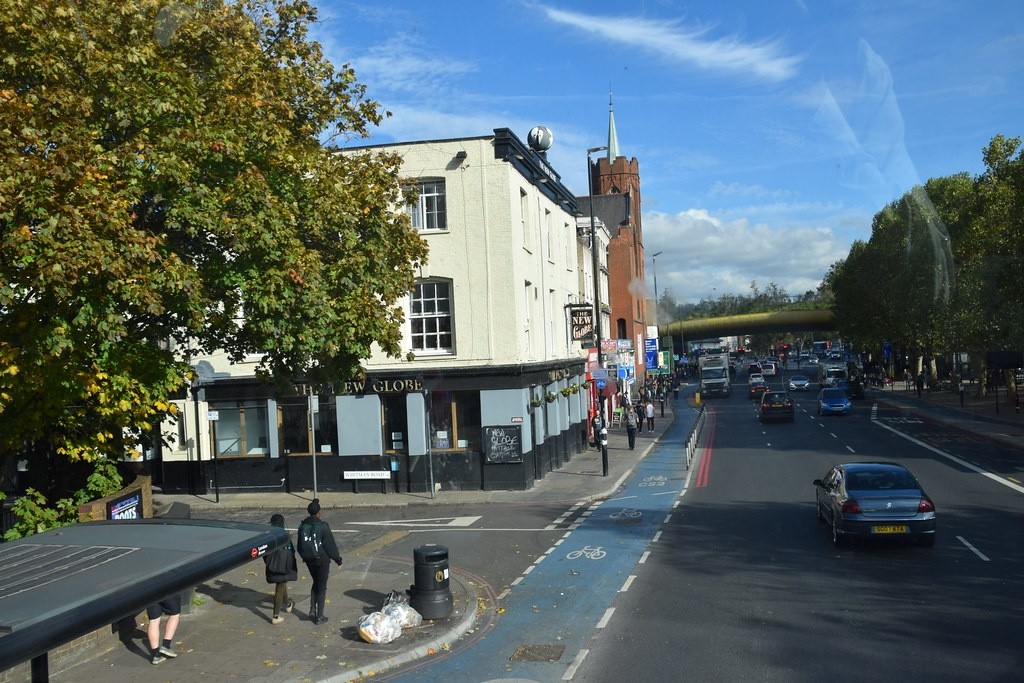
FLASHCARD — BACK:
[457,150,467,161]
[562,198,570,204]
[511,152,526,159]
[536,178,547,183]
[575,211,585,216]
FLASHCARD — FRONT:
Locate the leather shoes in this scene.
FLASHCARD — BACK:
[308,609,317,623]
[315,615,328,624]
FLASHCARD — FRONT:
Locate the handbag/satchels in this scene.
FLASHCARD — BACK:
[267,544,294,575]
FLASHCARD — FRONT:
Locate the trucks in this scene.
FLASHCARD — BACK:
[698,353,731,398]
[818,363,846,386]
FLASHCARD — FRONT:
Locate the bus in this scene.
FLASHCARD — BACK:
[812,341,826,351]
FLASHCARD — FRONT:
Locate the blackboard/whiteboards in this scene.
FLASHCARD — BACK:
[482,425,523,464]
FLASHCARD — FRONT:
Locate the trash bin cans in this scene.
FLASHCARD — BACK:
[410,544,454,620]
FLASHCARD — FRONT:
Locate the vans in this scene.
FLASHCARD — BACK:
[831,349,841,359]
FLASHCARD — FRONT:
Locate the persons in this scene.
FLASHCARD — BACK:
[969,368,976,386]
[592,411,606,453]
[638,357,702,408]
[861,360,887,390]
[634,402,646,432]
[903,369,912,391]
[623,405,640,450]
[986,368,994,393]
[622,392,629,414]
[260,514,298,625]
[146,593,182,664]
[645,401,655,433]
[297,499,342,625]
[915,372,926,398]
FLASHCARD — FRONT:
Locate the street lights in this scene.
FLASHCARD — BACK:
[587,146,608,476]
[652,252,662,352]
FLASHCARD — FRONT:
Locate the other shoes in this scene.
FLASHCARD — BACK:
[286,601,295,613]
[151,655,168,664]
[271,616,284,624]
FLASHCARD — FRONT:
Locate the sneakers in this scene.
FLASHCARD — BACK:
[158,645,178,658]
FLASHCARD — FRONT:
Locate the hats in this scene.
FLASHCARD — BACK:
[308,499,320,514]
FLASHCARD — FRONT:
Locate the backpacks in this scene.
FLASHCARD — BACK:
[626,414,637,426]
[592,417,601,429]
[298,524,325,563]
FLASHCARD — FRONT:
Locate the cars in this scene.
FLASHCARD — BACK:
[817,388,852,416]
[756,391,794,422]
[813,462,936,546]
[748,382,772,399]
[788,375,810,391]
[830,379,863,399]
[801,351,820,365]
[747,357,780,385]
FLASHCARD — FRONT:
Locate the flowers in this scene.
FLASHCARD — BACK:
[545,391,557,400]
[581,381,590,387]
[568,385,579,391]
[530,399,545,409]
[561,388,573,394]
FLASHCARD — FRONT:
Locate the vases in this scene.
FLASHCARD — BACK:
[584,385,588,389]
[572,390,577,394]
[546,398,555,403]
[563,392,569,397]
[531,402,540,407]
